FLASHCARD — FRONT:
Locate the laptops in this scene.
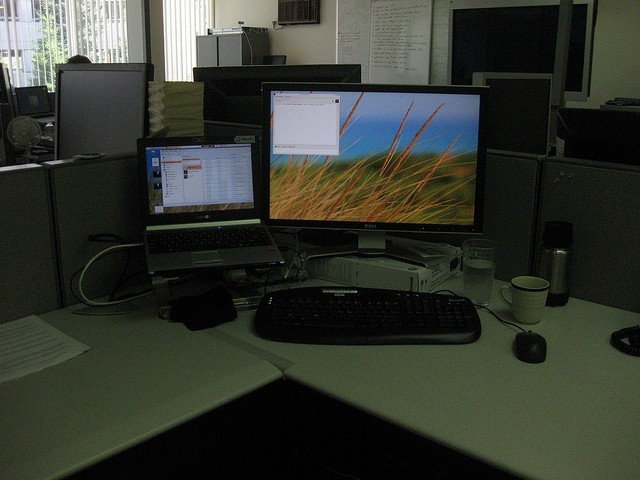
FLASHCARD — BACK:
[137,132,286,277]
[14,85,54,119]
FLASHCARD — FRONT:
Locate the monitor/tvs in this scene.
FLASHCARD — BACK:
[53,64,154,160]
[261,80,490,267]
[193,63,361,202]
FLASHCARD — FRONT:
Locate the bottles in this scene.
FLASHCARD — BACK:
[45,121,54,138]
[534,220,574,307]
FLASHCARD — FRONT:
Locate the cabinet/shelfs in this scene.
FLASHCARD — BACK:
[196,33,270,66]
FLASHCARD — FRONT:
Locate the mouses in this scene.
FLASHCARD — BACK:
[513,328,548,363]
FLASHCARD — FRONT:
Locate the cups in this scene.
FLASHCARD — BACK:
[462,238,498,311]
[497,276,551,325]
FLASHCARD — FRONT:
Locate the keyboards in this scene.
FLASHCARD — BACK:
[251,284,483,346]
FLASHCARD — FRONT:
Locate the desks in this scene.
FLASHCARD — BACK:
[0,271,639,480]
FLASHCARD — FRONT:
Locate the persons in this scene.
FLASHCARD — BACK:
[65,54,91,64]
